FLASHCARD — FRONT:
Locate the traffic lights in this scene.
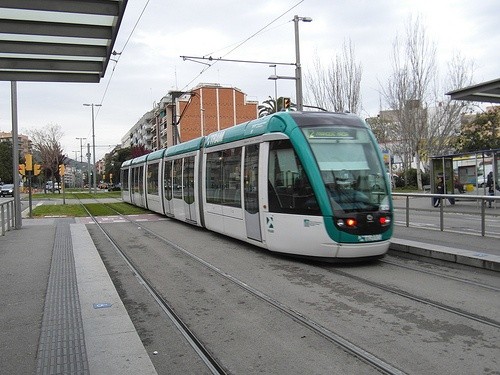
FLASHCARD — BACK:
[59,164,65,176]
[24,154,33,171]
[18,164,25,175]
[34,164,42,176]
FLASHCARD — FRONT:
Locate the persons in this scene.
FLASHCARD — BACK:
[295,181,308,202]
[454,177,465,193]
[434,177,447,207]
[487,172,494,196]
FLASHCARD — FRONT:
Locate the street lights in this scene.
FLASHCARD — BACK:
[76,138,86,188]
[268,15,313,112]
[84,104,102,195]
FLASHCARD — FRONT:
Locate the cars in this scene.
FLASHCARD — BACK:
[98,183,109,189]
[46,180,61,191]
[107,185,121,191]
[0,184,14,197]
[165,183,185,190]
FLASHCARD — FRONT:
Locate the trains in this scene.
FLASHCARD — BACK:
[120,112,394,258]
